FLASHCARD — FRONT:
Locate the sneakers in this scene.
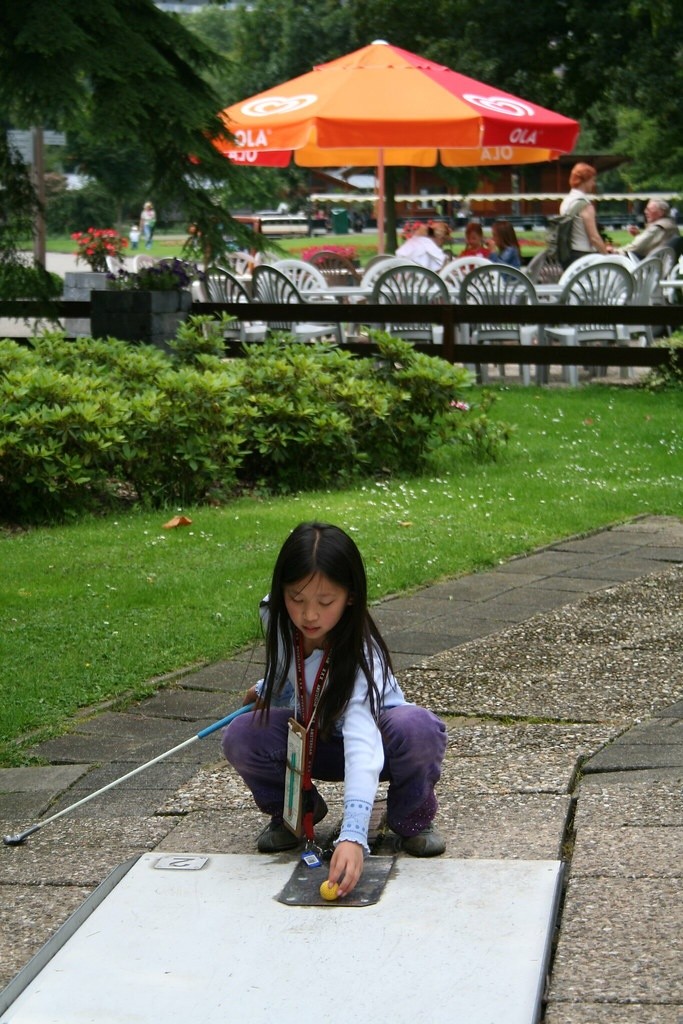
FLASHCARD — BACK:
[378,816,446,858]
[259,792,328,853]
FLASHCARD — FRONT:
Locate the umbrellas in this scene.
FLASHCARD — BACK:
[189,40,580,255]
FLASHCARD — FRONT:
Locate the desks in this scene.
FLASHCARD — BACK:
[220,280,683,297]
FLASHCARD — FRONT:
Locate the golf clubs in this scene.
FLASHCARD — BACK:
[2,701,256,845]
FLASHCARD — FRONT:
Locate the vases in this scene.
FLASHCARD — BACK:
[90,290,151,345]
[64,271,108,338]
[150,290,191,347]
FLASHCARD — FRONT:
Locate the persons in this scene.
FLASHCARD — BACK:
[129,225,142,251]
[316,209,333,234]
[457,222,490,259]
[488,221,521,285]
[222,520,446,897]
[606,198,681,258]
[140,202,156,249]
[559,162,609,261]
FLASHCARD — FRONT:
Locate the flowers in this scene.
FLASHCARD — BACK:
[105,268,143,291]
[116,256,207,291]
[72,227,130,273]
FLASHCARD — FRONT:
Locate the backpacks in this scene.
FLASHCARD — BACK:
[544,200,589,269]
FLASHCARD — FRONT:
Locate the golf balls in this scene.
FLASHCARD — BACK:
[319,879,340,901]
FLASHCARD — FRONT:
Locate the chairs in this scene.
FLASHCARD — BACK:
[186,246,675,386]
